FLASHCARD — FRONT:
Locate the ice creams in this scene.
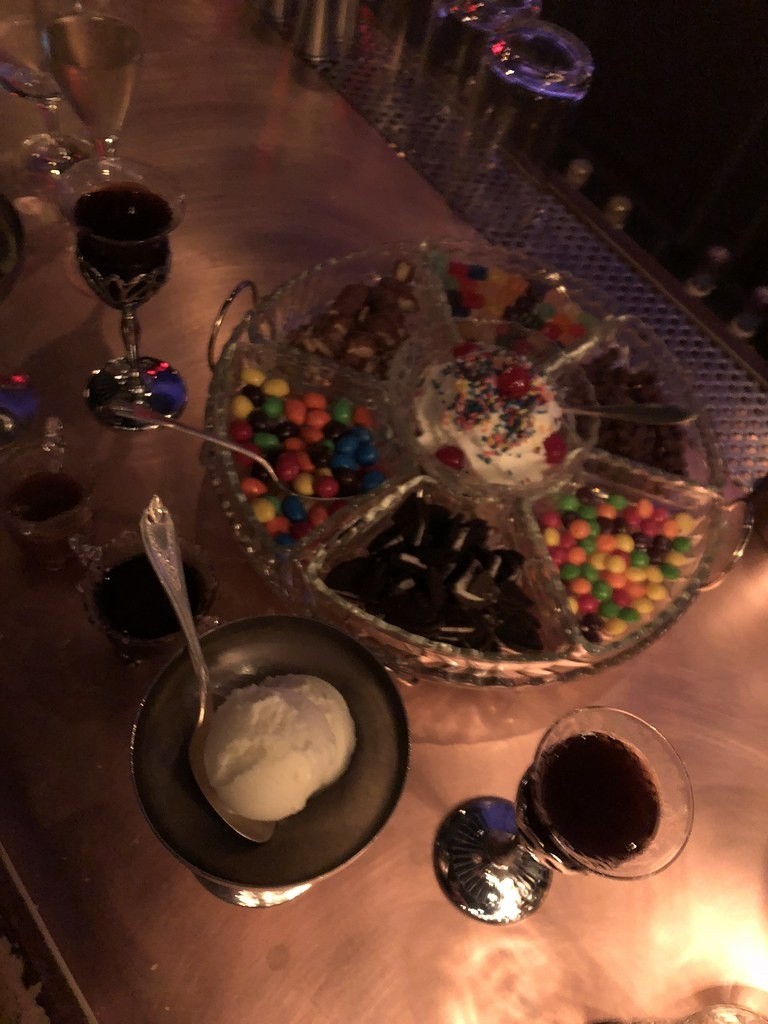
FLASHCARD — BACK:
[202,673,357,823]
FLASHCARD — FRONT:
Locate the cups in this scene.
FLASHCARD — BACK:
[130,615,411,910]
[365,0,442,120]
[405,0,541,174]
[262,0,360,64]
[444,16,593,234]
[0,416,98,573]
[71,522,219,658]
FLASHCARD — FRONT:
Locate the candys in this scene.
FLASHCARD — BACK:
[231,254,707,661]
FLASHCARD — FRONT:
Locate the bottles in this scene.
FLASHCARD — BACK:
[728,286,768,339]
[563,156,592,190]
[602,195,632,230]
[683,246,729,297]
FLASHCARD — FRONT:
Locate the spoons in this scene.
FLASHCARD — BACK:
[109,399,360,501]
[141,492,282,847]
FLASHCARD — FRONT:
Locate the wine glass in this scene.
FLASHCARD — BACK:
[0,190,40,437]
[432,702,695,924]
[0,0,76,163]
[52,156,188,430]
[40,12,154,193]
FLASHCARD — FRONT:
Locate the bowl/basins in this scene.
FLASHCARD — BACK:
[204,242,756,686]
